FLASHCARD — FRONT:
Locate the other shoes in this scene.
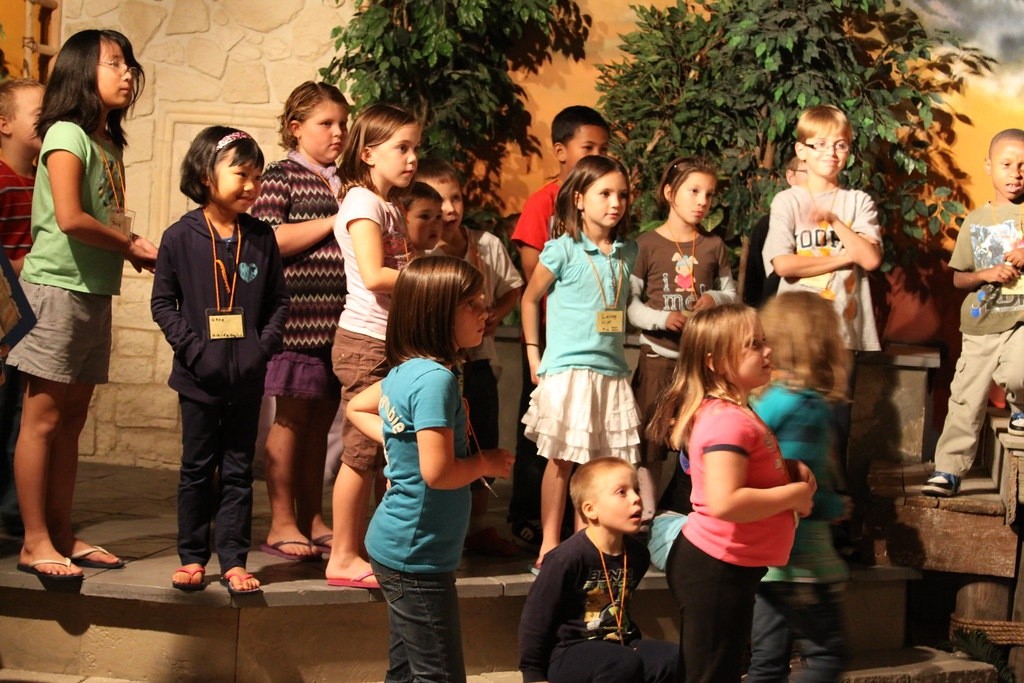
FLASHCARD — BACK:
[465,527,517,556]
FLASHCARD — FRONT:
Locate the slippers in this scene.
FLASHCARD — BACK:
[328,571,382,588]
[68,545,125,569]
[173,566,205,591]
[527,559,542,577]
[257,537,323,562]
[16,557,84,581]
[314,533,334,553]
[220,569,261,594]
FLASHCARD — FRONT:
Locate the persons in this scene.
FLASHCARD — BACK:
[1,26,158,585]
[918,128,1024,496]
[518,455,652,683]
[664,292,846,682]
[367,258,512,683]
[764,104,885,487]
[252,81,519,590]
[518,106,644,579]
[152,125,290,591]
[626,158,740,511]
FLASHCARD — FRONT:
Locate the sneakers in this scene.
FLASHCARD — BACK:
[511,520,543,552]
[923,470,961,496]
[1008,411,1024,437]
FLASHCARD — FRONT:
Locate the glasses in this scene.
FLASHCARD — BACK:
[98,58,138,82]
[800,138,852,154]
